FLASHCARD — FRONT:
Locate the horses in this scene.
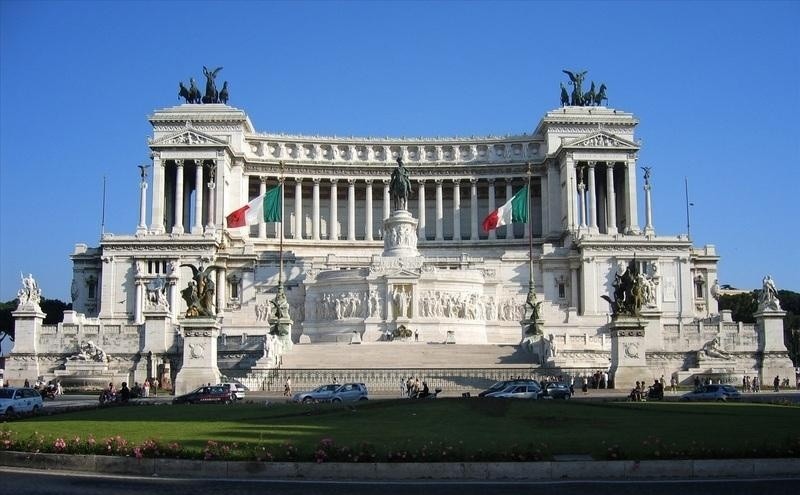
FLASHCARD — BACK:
[561,81,608,106]
[177,76,229,104]
[393,167,409,211]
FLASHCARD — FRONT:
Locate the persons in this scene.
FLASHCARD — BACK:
[694,375,720,390]
[762,274,778,298]
[333,377,339,384]
[228,273,238,299]
[414,329,419,341]
[629,375,677,401]
[743,376,791,392]
[569,370,609,396]
[107,377,159,402]
[510,374,564,399]
[554,64,610,109]
[180,66,229,103]
[78,340,106,358]
[284,376,291,396]
[23,378,64,401]
[400,376,429,399]
[608,266,646,317]
[145,272,165,302]
[385,328,391,341]
[387,157,415,197]
[85,275,96,299]
[186,265,216,313]
[202,383,210,387]
[15,270,42,306]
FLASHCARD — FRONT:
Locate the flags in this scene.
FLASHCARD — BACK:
[226,182,281,229]
[483,181,529,232]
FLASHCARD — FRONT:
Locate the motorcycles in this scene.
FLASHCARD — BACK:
[647,385,663,400]
[628,388,647,402]
[41,387,57,400]
[98,389,117,402]
[129,388,144,399]
[408,387,442,399]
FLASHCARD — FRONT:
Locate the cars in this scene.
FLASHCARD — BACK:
[679,385,741,404]
[328,382,369,402]
[293,384,346,404]
[484,385,543,401]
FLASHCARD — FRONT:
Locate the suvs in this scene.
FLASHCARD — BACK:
[217,382,245,401]
[0,388,43,416]
[171,386,233,404]
[478,378,540,397]
[540,381,571,400]
